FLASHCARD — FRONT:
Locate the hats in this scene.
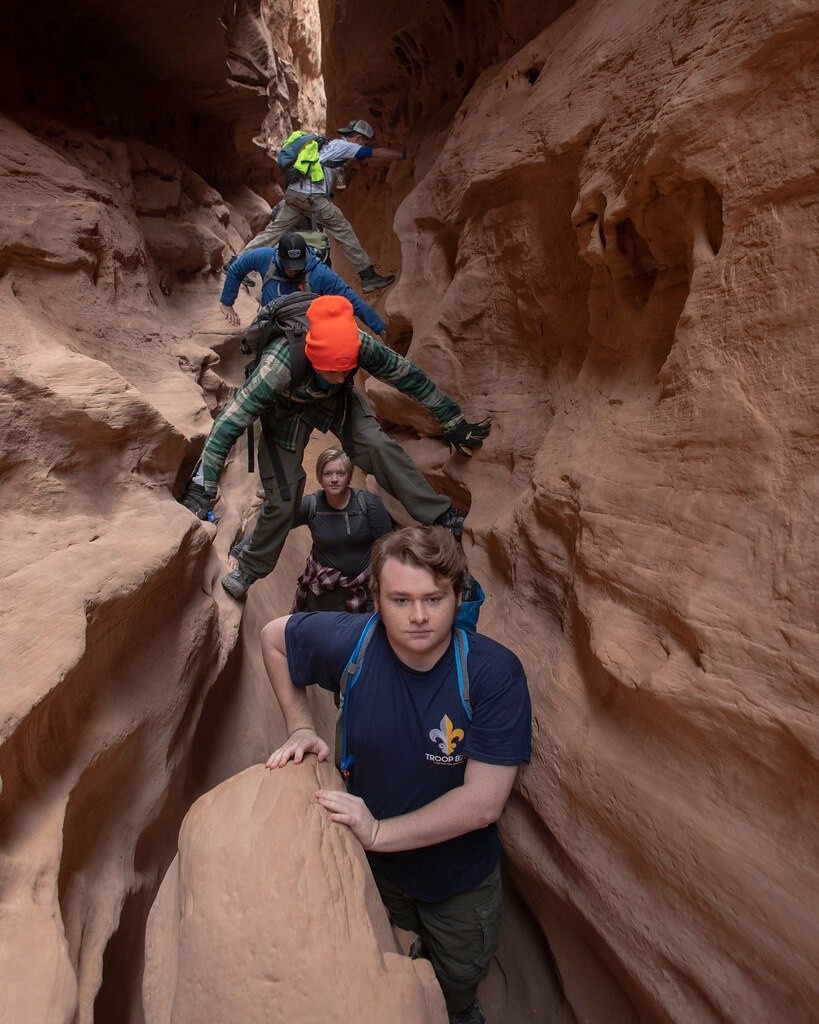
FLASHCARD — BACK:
[336,119,375,140]
[304,295,361,371]
[278,234,307,270]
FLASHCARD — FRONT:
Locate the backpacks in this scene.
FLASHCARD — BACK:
[333,572,485,777]
[259,230,332,291]
[239,291,365,501]
[277,130,351,183]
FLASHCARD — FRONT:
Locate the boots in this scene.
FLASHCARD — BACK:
[222,255,256,287]
[358,265,395,294]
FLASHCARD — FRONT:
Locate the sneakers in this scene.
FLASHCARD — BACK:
[444,508,467,538]
[221,568,258,599]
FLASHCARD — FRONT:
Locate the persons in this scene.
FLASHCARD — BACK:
[227,446,395,616]
[220,231,391,348]
[187,294,493,600]
[221,118,422,293]
[269,199,314,233]
[259,527,535,1023]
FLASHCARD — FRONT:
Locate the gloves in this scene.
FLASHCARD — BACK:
[177,483,221,520]
[440,412,492,457]
